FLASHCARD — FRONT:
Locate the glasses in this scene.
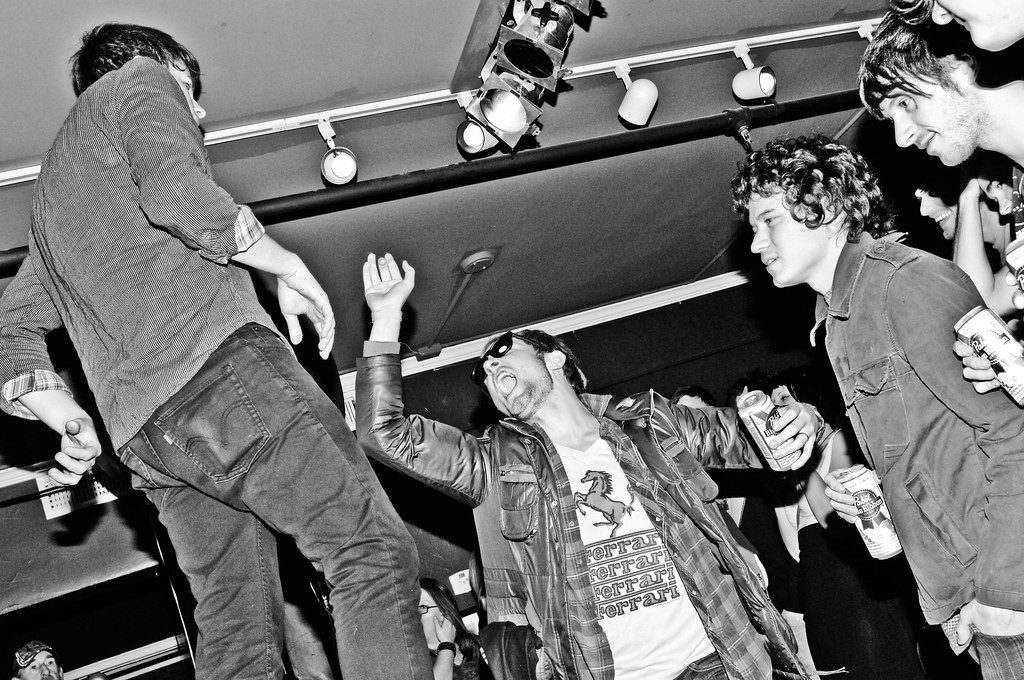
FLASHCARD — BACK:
[416,605,440,614]
[470,331,552,400]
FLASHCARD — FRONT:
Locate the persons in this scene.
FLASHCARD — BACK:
[856,0,1023,405]
[729,135,1024,680]
[360,252,982,680]
[9,642,67,680]
[0,21,435,680]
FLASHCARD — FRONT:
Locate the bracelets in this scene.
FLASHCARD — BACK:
[436,642,457,659]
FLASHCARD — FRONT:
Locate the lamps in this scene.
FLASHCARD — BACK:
[731,45,777,100]
[316,121,358,186]
[613,62,659,126]
[456,0,595,155]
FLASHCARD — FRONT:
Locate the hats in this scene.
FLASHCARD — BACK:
[12,640,59,674]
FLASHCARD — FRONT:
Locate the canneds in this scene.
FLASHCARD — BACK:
[953,305,1024,407]
[737,390,802,472]
[1004,235,1024,296]
[836,464,903,560]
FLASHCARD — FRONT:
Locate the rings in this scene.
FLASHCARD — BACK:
[800,433,810,441]
